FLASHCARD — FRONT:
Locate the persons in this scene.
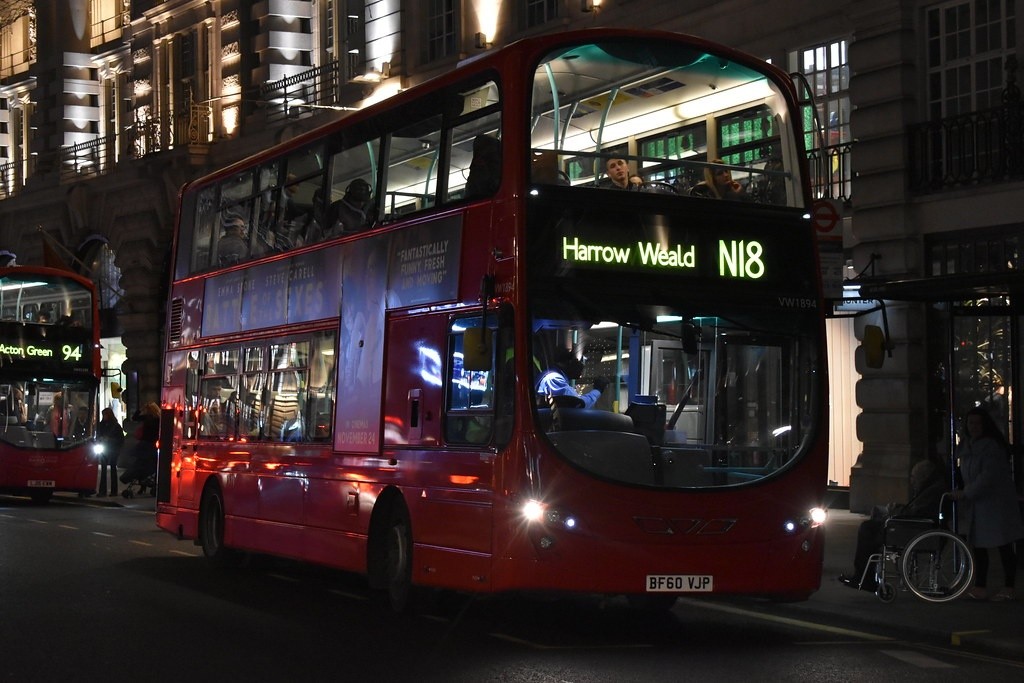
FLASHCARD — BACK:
[5,389,26,424]
[206,381,226,403]
[217,180,373,264]
[132,402,161,496]
[943,406,1024,601]
[605,150,648,194]
[69,406,92,440]
[839,460,947,594]
[704,157,752,201]
[44,392,69,443]
[37,313,51,324]
[95,408,123,497]
[533,352,611,411]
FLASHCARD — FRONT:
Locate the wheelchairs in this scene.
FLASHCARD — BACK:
[858,492,974,605]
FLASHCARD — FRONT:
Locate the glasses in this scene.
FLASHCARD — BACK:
[232,225,248,230]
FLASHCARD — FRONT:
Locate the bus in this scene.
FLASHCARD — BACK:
[0,250,122,504]
[156,26,895,614]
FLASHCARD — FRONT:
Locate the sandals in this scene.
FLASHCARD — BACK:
[968,590,986,600]
[991,590,1016,601]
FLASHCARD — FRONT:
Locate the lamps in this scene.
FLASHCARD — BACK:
[472,0,502,48]
[581,0,603,17]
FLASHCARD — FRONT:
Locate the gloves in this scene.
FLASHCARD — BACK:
[593,376,610,393]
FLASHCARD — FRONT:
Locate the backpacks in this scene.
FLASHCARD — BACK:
[55,315,74,326]
[755,157,788,206]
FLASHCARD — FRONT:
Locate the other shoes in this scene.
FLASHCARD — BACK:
[839,574,877,592]
[109,493,117,496]
[97,493,106,496]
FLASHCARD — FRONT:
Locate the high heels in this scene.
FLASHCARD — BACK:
[150,488,155,495]
[138,485,146,494]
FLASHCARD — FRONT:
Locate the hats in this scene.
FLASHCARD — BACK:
[314,188,332,205]
[13,382,24,393]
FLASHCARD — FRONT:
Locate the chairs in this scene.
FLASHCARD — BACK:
[220,385,333,441]
[552,170,681,196]
